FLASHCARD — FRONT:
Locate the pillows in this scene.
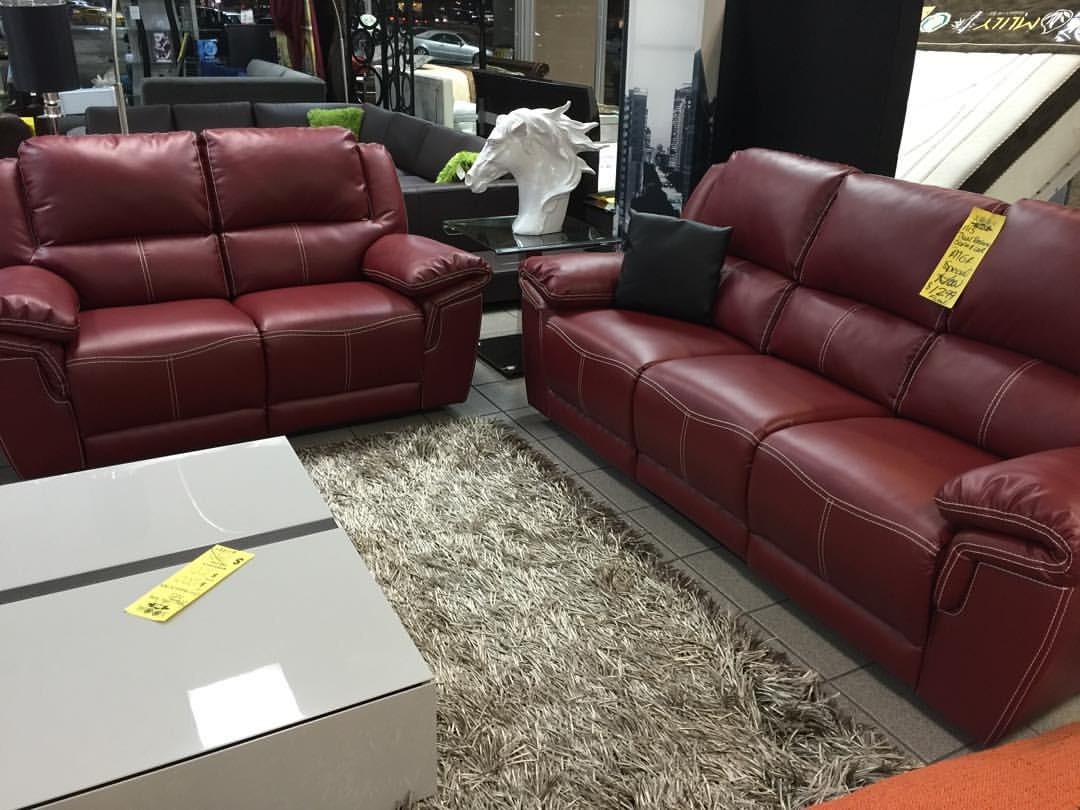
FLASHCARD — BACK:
[436,151,480,184]
[307,104,364,138]
[615,203,732,329]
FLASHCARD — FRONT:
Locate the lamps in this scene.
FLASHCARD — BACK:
[0,3,81,116]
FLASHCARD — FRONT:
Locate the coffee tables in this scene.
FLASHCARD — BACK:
[0,433,440,810]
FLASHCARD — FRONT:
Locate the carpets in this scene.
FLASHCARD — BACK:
[281,418,917,809]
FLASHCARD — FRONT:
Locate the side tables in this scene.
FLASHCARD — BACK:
[444,215,621,383]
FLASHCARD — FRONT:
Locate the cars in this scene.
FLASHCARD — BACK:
[122,6,242,54]
[400,30,494,68]
[70,0,126,27]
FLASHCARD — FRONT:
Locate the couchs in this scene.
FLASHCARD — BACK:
[520,149,1080,753]
[141,58,327,103]
[1,126,493,480]
[86,103,520,243]
